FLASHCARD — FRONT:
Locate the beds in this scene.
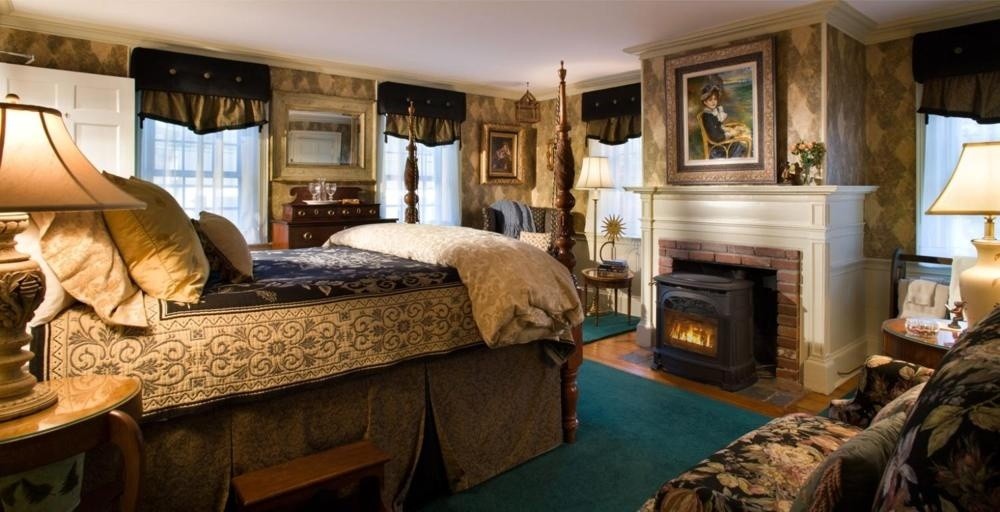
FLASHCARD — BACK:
[24,58,584,499]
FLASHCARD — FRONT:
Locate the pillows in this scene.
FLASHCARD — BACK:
[869,382,929,420]
[199,210,253,284]
[30,210,150,330]
[99,169,210,304]
[14,217,77,329]
[788,411,905,510]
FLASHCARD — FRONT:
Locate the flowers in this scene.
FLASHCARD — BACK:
[791,139,827,165]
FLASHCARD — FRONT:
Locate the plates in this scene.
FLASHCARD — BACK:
[303,199,341,205]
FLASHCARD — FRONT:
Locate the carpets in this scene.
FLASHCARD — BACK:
[583,310,641,343]
[318,355,775,512]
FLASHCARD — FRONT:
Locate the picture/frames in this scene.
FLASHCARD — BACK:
[478,122,526,186]
[663,33,778,186]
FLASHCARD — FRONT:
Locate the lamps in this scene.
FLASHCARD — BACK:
[923,141,1000,328]
[0,91,150,424]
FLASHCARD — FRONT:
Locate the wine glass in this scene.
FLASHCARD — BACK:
[309,181,322,202]
[326,182,336,200]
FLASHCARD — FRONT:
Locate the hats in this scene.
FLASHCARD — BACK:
[700,83,721,102]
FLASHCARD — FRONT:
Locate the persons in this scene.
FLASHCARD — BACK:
[701,84,751,158]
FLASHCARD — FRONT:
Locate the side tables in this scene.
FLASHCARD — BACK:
[581,267,634,325]
[881,316,967,368]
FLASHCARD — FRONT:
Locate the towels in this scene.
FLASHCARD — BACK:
[901,280,950,321]
[908,278,936,305]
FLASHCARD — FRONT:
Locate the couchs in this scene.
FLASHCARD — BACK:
[636,304,1000,512]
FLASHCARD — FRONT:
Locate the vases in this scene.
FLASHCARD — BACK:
[804,165,824,184]
